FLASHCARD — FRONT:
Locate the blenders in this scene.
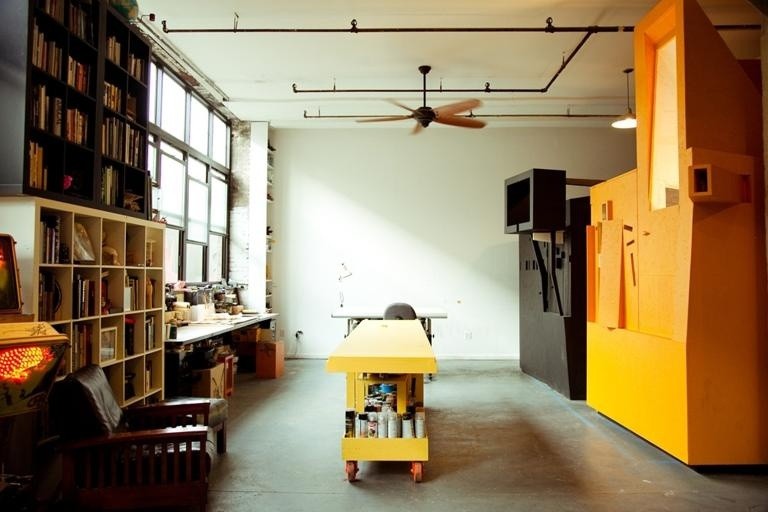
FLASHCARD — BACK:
[165,283,177,327]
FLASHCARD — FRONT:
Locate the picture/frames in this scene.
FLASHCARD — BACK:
[0,233,24,314]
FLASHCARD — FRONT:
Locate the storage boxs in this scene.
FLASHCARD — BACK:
[255,341,284,378]
[191,363,224,399]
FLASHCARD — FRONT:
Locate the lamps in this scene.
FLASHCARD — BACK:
[610,68,637,129]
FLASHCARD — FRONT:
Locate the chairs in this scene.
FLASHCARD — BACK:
[52,364,215,512]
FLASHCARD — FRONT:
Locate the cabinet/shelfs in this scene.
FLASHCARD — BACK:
[251,121,274,313]
[504,168,590,400]
[0,1,154,221]
[586,0,768,466]
[0,196,166,408]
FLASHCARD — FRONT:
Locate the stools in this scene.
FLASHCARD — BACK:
[152,396,228,454]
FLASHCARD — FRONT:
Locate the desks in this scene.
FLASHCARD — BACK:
[331,313,448,382]
[164,313,279,365]
[327,318,438,482]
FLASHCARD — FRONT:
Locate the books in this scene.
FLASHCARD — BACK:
[51,313,156,378]
[102,117,145,171]
[38,2,98,50]
[32,17,90,96]
[100,165,144,212]
[106,36,145,83]
[102,353,153,401]
[29,140,48,191]
[38,270,156,321]
[103,80,137,123]
[38,215,154,267]
[31,83,89,148]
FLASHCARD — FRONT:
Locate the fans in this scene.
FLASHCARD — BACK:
[355,65,487,136]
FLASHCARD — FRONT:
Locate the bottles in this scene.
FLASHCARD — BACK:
[166,324,177,340]
[174,303,190,322]
[345,407,425,438]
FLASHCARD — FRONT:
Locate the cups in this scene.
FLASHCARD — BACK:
[190,303,216,322]
